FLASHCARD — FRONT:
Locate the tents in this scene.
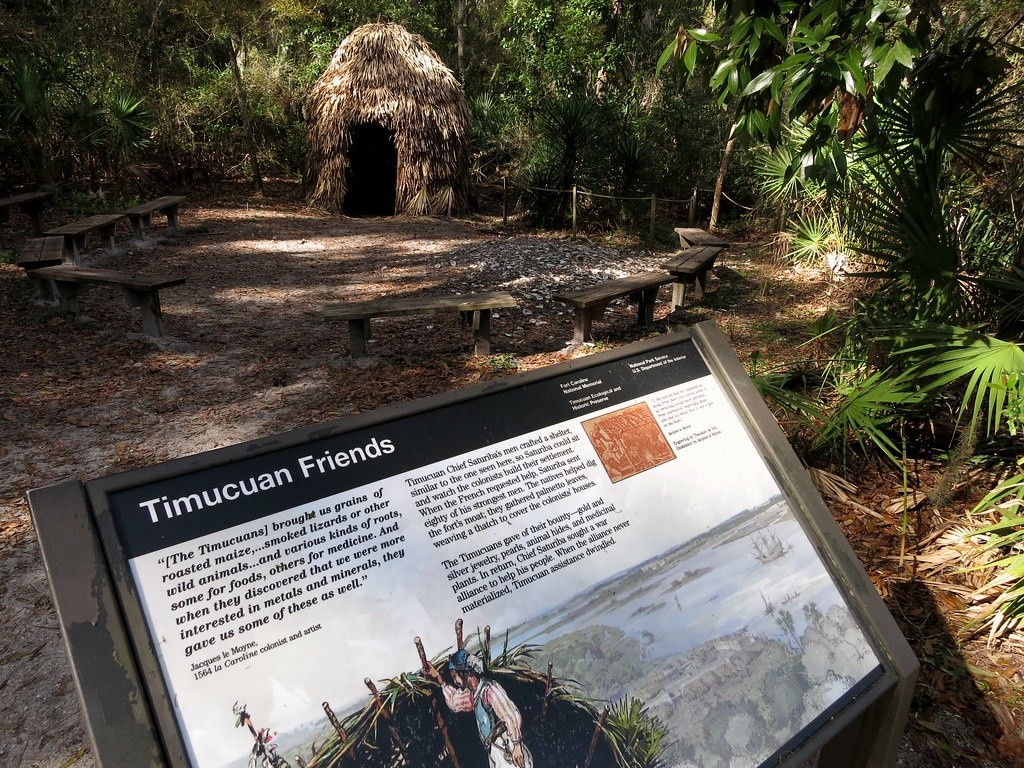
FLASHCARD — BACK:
[302,21,479,217]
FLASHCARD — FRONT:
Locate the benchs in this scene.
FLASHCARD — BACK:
[42,213,126,266]
[0,192,55,248]
[663,245,724,311]
[121,195,186,236]
[322,290,517,359]
[550,271,679,344]
[674,227,730,285]
[28,265,187,338]
[17,235,66,290]
